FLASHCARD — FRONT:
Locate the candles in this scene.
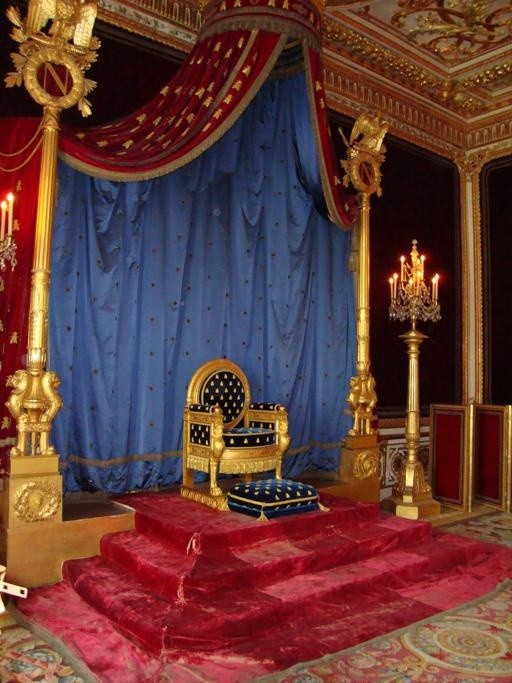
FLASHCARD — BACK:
[0,188,16,245]
[386,251,441,303]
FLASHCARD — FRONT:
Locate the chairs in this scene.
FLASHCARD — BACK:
[179,358,293,511]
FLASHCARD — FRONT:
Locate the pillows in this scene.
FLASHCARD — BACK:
[220,476,330,520]
